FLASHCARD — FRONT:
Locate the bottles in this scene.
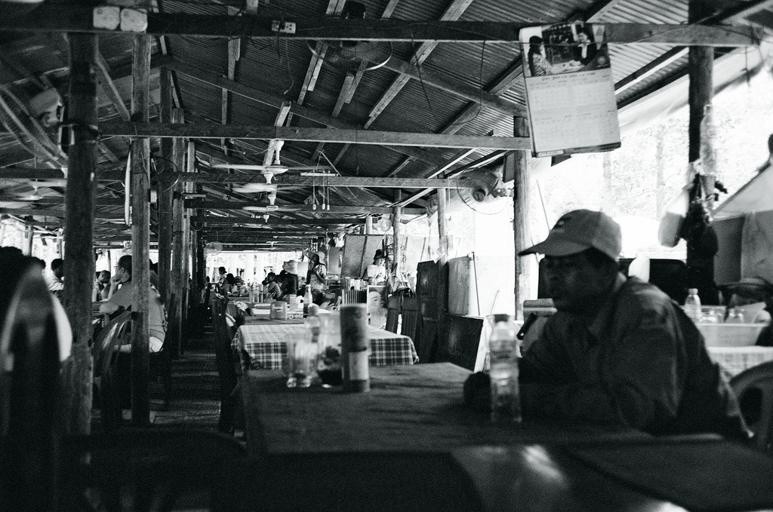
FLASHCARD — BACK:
[488,313,523,425]
[239,279,272,302]
[289,293,297,310]
[725,309,745,324]
[302,284,313,318]
[699,104,719,172]
[315,312,343,388]
[683,288,700,322]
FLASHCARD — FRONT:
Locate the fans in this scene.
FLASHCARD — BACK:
[150,155,180,196]
[305,0,393,72]
[214,140,328,223]
[456,168,516,216]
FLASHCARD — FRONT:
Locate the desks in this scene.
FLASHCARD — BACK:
[450,443,773,512]
[230,362,689,512]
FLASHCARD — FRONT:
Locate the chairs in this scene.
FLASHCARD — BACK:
[93,284,184,430]
[209,281,427,423]
[730,361,773,456]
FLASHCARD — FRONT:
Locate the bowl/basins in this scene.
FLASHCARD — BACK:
[694,323,768,347]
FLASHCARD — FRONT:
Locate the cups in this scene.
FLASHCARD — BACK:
[283,334,313,388]
[338,304,369,391]
[269,301,286,320]
[307,304,318,315]
[700,307,724,326]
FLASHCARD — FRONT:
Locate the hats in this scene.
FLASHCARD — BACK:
[517,209,622,265]
[309,251,326,265]
[283,260,298,275]
[373,250,389,263]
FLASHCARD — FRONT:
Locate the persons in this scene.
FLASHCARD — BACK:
[364,250,389,286]
[512,208,751,446]
[262,259,299,302]
[527,27,610,75]
[368,286,388,329]
[305,251,327,307]
[2,245,168,377]
[218,266,244,296]
